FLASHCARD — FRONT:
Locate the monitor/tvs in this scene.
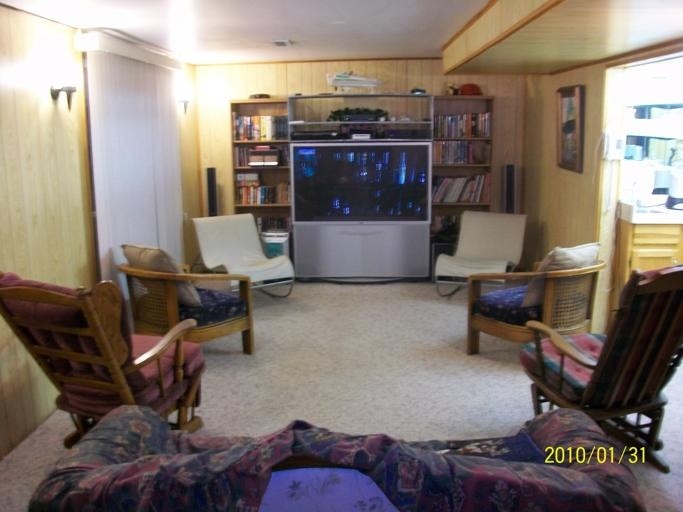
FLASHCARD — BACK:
[289,142,432,222]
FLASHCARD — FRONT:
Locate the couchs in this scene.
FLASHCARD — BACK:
[26,405,646,511]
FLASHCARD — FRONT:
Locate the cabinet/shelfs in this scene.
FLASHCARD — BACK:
[431,96,494,242]
[288,94,434,142]
[229,96,291,283]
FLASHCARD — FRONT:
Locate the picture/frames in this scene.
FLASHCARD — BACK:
[555,85,585,175]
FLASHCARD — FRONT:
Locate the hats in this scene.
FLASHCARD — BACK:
[461,83,482,95]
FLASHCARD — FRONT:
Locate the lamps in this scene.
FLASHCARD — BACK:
[49,54,77,114]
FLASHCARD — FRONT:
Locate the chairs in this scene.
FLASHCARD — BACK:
[112,244,256,356]
[521,263,682,472]
[464,240,608,358]
[189,211,296,301]
[430,210,530,298]
[0,270,208,451]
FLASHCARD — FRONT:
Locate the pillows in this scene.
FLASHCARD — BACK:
[521,240,602,310]
[121,245,203,307]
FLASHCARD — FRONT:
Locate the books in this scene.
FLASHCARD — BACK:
[232,112,291,233]
[432,112,491,203]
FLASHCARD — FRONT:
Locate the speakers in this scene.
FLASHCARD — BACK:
[502,163,517,215]
[206,168,218,217]
